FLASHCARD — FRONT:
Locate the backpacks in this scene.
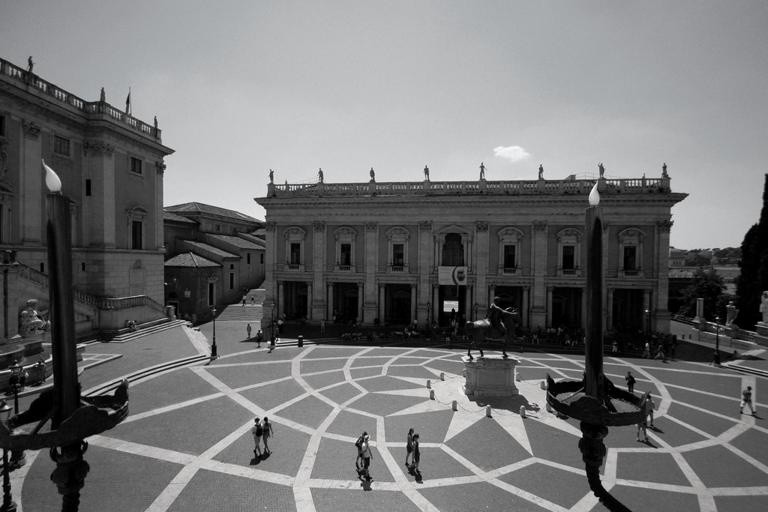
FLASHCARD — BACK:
[355,436,363,446]
[255,423,263,436]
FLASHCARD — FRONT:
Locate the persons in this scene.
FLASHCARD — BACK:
[268,313,287,353]
[243,294,246,306]
[355,432,372,480]
[761,290,768,323]
[726,301,737,324]
[625,372,636,393]
[636,395,655,443]
[21,299,45,337]
[547,327,579,347]
[320,309,363,341]
[257,330,262,347]
[489,296,519,338]
[129,320,134,328]
[642,340,675,362]
[611,339,618,353]
[9,358,47,393]
[252,417,273,456]
[405,428,421,474]
[741,387,756,414]
[247,324,251,338]
[527,326,541,344]
[404,316,460,345]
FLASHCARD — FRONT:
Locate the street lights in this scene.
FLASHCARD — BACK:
[713,316,720,364]
[644,307,651,335]
[211,306,217,355]
[0,399,17,512]
[8,359,24,414]
[270,301,275,345]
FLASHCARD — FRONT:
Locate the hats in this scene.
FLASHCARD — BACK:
[364,435,371,442]
